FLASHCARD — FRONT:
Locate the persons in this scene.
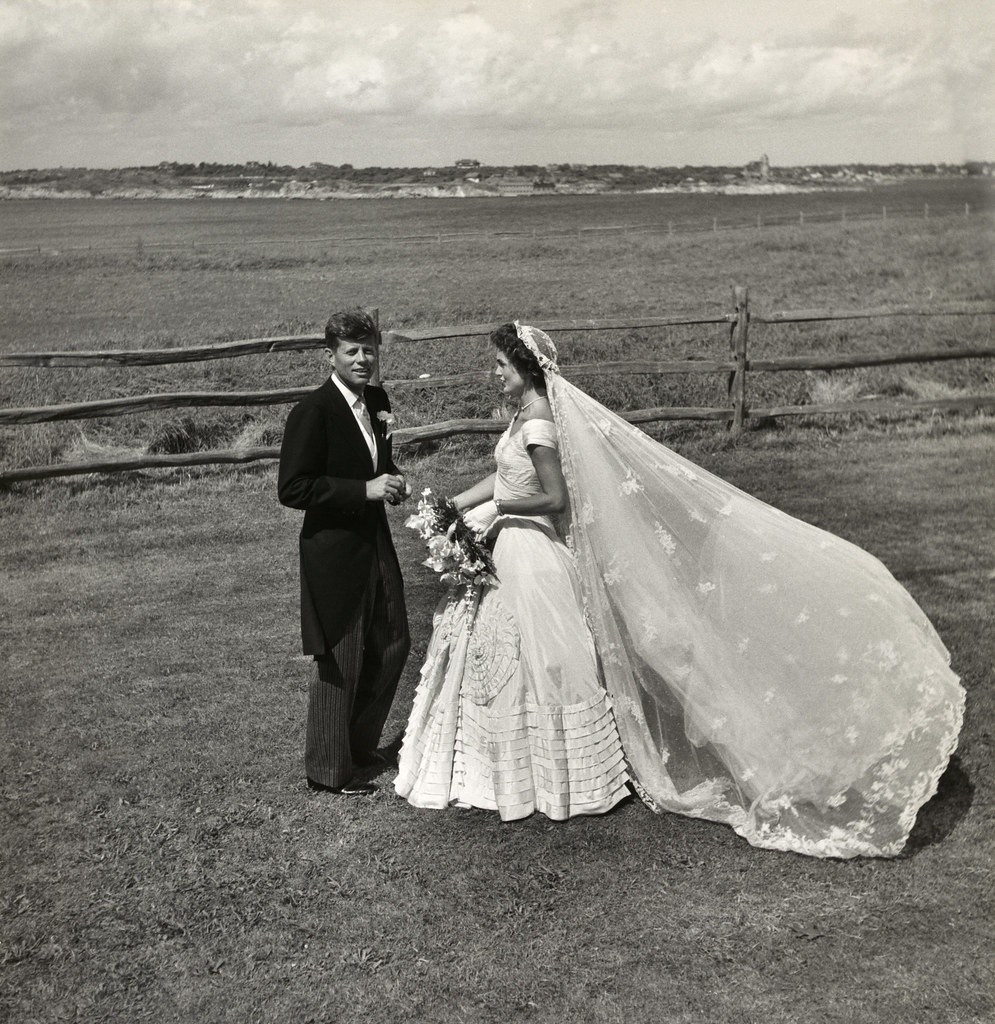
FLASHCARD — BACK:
[390,320,967,859]
[277,306,414,797]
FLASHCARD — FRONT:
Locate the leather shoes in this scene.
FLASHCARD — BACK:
[307,777,377,796]
[352,749,393,776]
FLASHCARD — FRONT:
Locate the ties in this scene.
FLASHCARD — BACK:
[358,397,374,444]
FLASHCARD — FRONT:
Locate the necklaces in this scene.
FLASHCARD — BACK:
[521,394,547,411]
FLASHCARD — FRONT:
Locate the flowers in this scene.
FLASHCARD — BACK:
[377,410,395,442]
[404,486,502,588]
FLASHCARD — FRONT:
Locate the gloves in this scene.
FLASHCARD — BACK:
[463,501,499,534]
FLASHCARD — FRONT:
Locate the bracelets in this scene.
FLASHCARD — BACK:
[497,499,504,516]
